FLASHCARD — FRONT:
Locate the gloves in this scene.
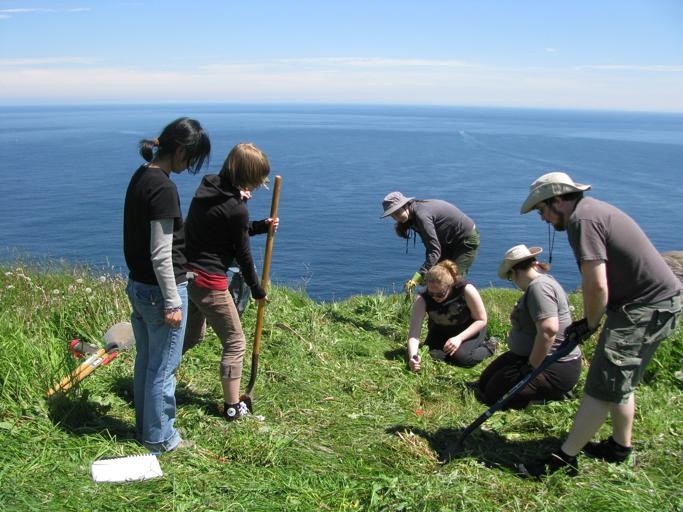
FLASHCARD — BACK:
[565,319,599,345]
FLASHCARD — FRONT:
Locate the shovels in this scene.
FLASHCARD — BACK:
[240,175,282,416]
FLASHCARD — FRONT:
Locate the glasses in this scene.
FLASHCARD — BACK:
[428,288,450,299]
[537,203,549,215]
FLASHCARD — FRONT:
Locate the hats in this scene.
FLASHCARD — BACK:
[519,171,592,215]
[380,190,414,218]
[498,244,542,280]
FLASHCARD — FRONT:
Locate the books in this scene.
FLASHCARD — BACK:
[89,453,163,483]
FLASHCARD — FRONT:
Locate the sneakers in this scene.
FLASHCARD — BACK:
[585,437,633,463]
[519,450,579,479]
[169,439,196,450]
[223,401,266,422]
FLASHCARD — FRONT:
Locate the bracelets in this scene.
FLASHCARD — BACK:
[163,306,182,312]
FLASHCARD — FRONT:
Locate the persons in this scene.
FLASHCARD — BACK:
[478,242,582,411]
[177,142,281,422]
[122,118,210,453]
[378,194,481,293]
[404,257,498,374]
[515,171,682,480]
